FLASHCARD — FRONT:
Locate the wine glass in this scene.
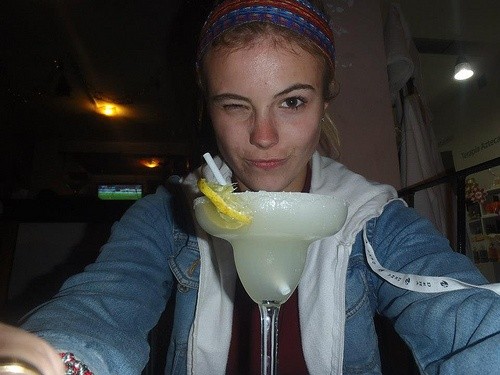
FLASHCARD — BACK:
[193,191,348,375]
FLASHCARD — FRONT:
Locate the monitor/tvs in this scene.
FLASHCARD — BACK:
[96,184,144,201]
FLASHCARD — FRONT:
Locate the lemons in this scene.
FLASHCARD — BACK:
[198,178,250,224]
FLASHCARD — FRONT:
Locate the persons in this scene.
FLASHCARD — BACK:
[14,0,499,375]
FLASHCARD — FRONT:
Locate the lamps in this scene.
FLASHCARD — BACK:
[453,57,475,82]
